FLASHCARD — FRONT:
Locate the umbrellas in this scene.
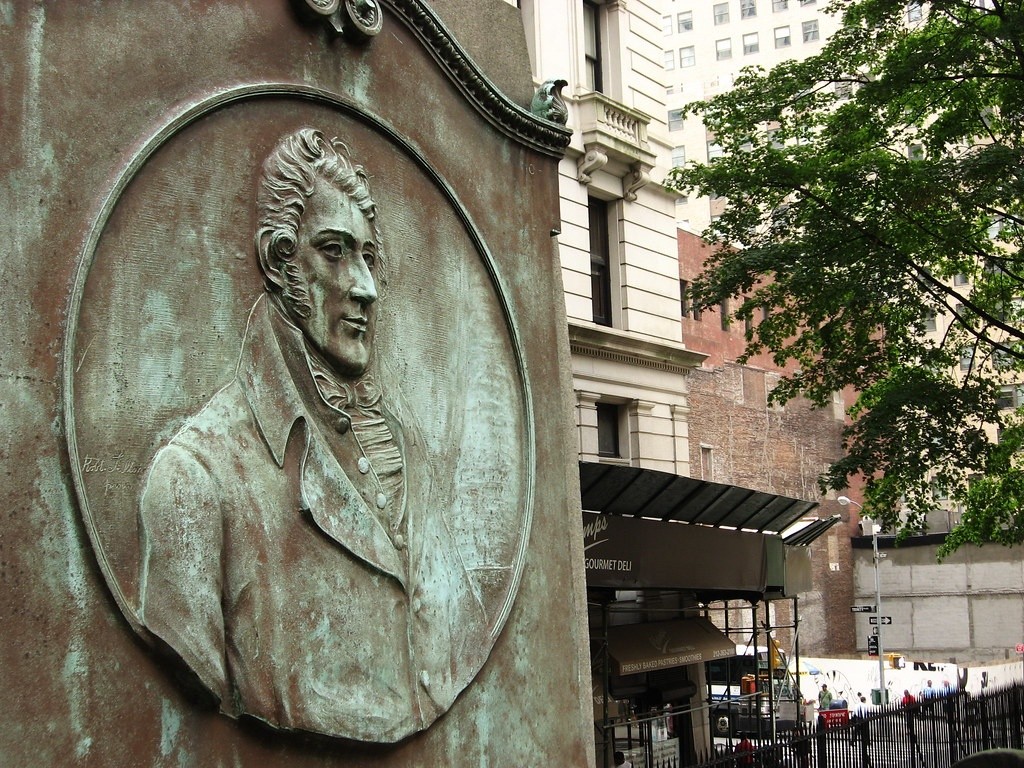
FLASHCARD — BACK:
[785,660,819,675]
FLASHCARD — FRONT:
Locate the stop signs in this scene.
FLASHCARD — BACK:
[1015,643,1024,654]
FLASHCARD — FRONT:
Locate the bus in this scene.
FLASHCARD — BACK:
[705,645,808,740]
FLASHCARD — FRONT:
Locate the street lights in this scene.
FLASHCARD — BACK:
[837,496,887,707]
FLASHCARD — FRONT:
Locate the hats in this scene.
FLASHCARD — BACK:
[821,684,827,687]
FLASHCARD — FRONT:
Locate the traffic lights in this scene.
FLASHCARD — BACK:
[891,655,906,670]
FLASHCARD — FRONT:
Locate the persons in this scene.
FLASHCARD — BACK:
[141,127,480,743]
[615,680,958,768]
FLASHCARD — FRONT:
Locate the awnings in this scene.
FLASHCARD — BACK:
[597,616,737,678]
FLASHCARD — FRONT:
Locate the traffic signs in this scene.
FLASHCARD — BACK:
[850,605,877,614]
[869,616,892,625]
